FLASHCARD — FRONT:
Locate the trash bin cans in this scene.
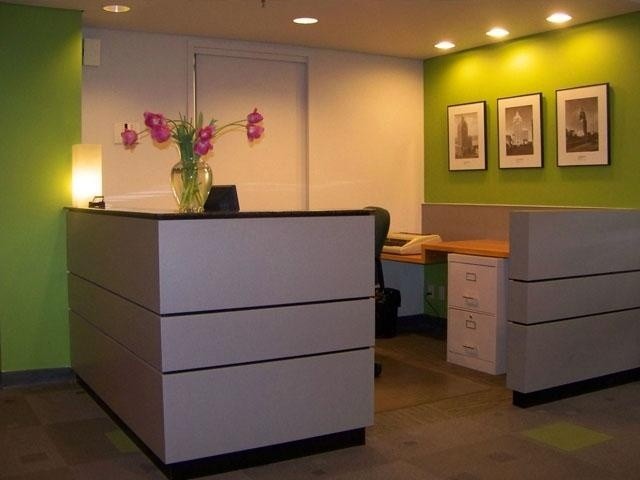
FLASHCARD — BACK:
[375,287,401,338]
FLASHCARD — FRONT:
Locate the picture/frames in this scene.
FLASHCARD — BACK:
[447,82,610,172]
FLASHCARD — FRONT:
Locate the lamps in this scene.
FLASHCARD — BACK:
[70,142,103,209]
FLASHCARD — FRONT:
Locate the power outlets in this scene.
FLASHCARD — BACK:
[427,285,434,298]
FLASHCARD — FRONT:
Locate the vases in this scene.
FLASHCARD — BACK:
[169,146,215,214]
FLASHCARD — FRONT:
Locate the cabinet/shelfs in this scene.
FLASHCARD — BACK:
[446,252,509,376]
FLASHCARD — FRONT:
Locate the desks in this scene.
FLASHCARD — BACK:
[378,237,509,266]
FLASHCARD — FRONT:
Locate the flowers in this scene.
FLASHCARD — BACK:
[121,107,265,208]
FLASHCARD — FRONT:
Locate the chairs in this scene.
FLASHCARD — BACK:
[359,205,401,379]
